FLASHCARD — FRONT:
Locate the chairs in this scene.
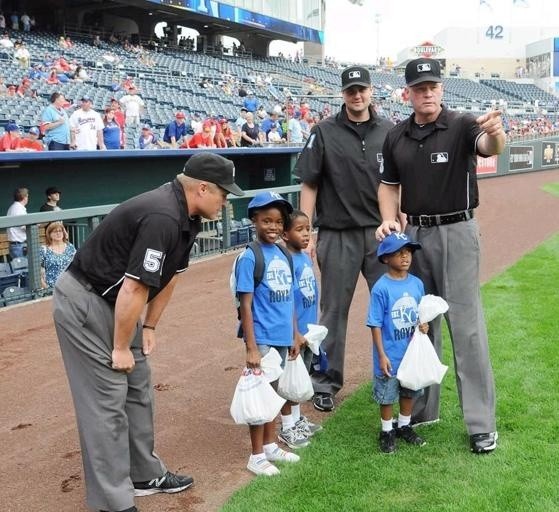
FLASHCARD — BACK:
[0,254,43,308]
[216,218,254,247]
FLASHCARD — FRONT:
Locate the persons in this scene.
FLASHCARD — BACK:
[277,210,322,449]
[507,115,557,142]
[162,71,328,150]
[53,151,244,511]
[4,188,32,261]
[148,33,195,52]
[217,41,245,58]
[366,234,430,453]
[57,35,141,55]
[39,222,77,296]
[278,50,338,68]
[377,59,507,454]
[235,188,302,479]
[2,53,159,152]
[0,11,31,67]
[295,68,405,410]
[39,187,65,231]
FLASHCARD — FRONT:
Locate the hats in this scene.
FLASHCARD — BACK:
[182,152,245,196]
[7,123,40,136]
[405,58,441,86]
[175,112,227,126]
[342,67,370,90]
[247,191,293,218]
[377,231,421,263]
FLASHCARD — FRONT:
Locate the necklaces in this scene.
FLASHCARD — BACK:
[188,214,196,222]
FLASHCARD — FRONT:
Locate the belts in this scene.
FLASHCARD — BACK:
[406,210,473,228]
[68,264,101,297]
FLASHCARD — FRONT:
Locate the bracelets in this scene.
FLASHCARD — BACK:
[143,325,156,330]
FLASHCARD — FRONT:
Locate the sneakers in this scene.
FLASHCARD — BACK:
[247,415,323,475]
[312,392,335,411]
[379,417,439,453]
[470,431,498,452]
[133,471,194,497]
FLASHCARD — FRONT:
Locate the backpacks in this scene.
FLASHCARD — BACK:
[230,241,293,320]
